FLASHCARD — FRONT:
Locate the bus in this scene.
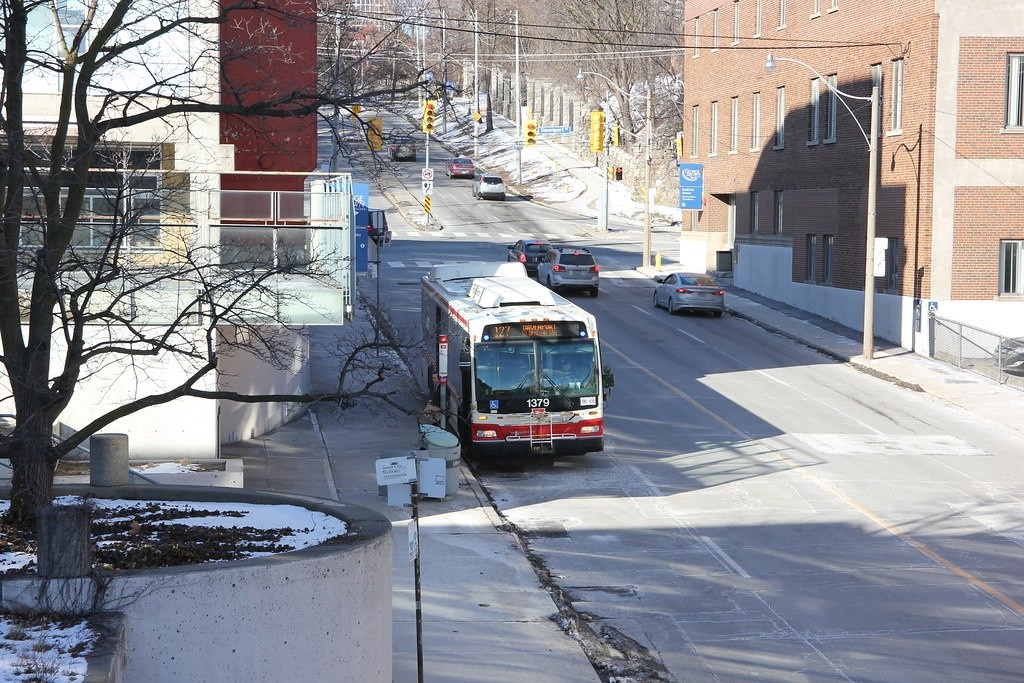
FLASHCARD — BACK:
[421,257,615,464]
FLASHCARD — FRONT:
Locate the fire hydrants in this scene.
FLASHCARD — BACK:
[655,252,663,271]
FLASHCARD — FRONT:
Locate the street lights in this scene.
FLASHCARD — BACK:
[576,68,650,267]
[472,64,522,186]
[391,39,422,111]
[765,54,878,358]
[431,53,479,157]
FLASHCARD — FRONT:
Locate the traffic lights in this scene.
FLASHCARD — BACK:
[525,121,536,146]
[616,167,623,180]
[673,137,683,159]
[611,127,619,147]
[423,100,435,133]
[606,167,614,180]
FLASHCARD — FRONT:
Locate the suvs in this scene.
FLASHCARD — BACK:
[535,246,600,299]
[386,137,416,162]
[507,239,555,274]
[471,174,505,201]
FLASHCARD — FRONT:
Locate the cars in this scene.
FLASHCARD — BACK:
[446,158,475,179]
[654,272,724,316]
[368,210,389,244]
